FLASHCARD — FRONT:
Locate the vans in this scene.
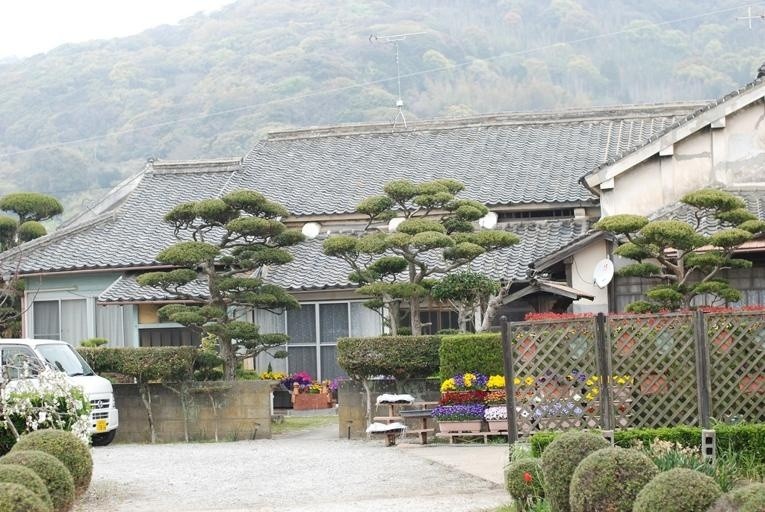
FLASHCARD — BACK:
[0,336,120,449]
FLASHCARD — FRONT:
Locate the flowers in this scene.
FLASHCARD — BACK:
[259,370,352,393]
[431,368,634,417]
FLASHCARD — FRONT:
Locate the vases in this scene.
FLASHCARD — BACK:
[486,419,530,432]
[584,414,632,428]
[538,416,580,430]
[438,418,482,433]
